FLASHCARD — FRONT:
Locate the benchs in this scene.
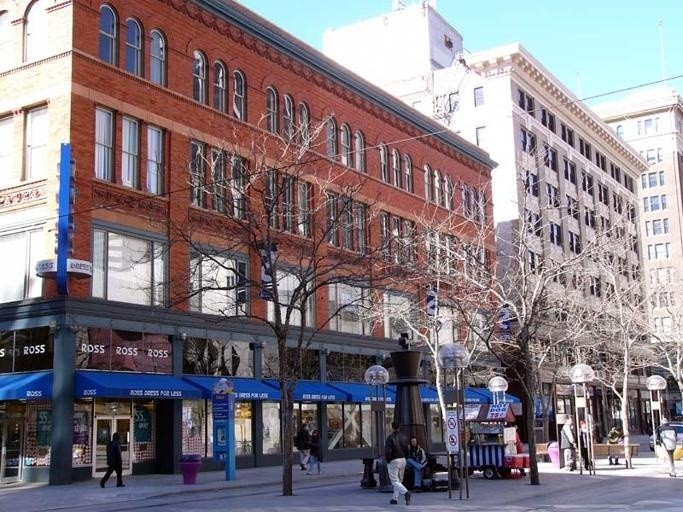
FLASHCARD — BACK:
[536,443,639,468]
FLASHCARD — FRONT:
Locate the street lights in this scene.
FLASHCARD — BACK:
[363,365,391,458]
[436,342,472,497]
[487,373,507,403]
[645,373,666,452]
[570,362,596,475]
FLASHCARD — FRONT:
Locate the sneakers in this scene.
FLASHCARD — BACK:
[117,485,125,487]
[390,500,398,504]
[300,462,306,470]
[100,481,105,488]
[406,492,411,505]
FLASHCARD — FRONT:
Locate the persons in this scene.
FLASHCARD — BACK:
[511,422,526,476]
[308,428,324,475]
[98,430,126,488]
[296,423,310,471]
[606,425,621,465]
[383,419,412,505]
[559,415,578,474]
[655,416,677,477]
[354,430,366,448]
[578,420,591,470]
[406,434,428,492]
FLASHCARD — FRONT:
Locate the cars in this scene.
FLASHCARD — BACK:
[645,422,682,455]
